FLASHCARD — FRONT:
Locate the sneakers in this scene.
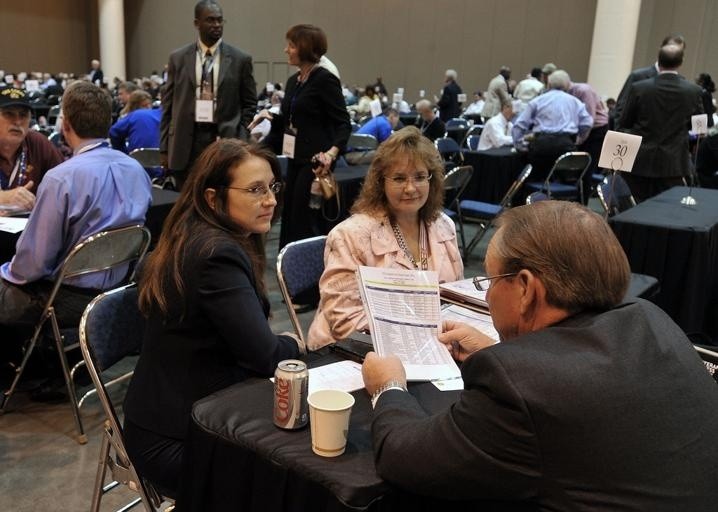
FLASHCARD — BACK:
[21,334,95,402]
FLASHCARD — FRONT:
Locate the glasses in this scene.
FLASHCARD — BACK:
[383,171,433,184]
[226,182,282,195]
[471,272,518,292]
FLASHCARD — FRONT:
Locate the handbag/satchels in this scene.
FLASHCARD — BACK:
[317,173,341,223]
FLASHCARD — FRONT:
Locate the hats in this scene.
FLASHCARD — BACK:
[0,86,33,110]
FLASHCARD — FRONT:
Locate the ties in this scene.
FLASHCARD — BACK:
[200,51,215,101]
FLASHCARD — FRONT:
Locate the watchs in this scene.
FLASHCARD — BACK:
[369,381,408,410]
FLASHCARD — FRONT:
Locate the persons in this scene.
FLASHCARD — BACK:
[0,59,166,213]
[0,84,154,399]
[161,0,257,194]
[247,23,353,316]
[605,36,716,204]
[320,54,404,152]
[462,62,608,207]
[435,69,463,122]
[305,124,465,353]
[413,100,445,142]
[362,199,718,511]
[251,82,287,145]
[117,138,330,511]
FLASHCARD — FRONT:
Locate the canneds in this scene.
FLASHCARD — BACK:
[273,359,309,429]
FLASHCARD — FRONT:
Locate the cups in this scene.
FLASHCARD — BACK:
[305,390,357,460]
